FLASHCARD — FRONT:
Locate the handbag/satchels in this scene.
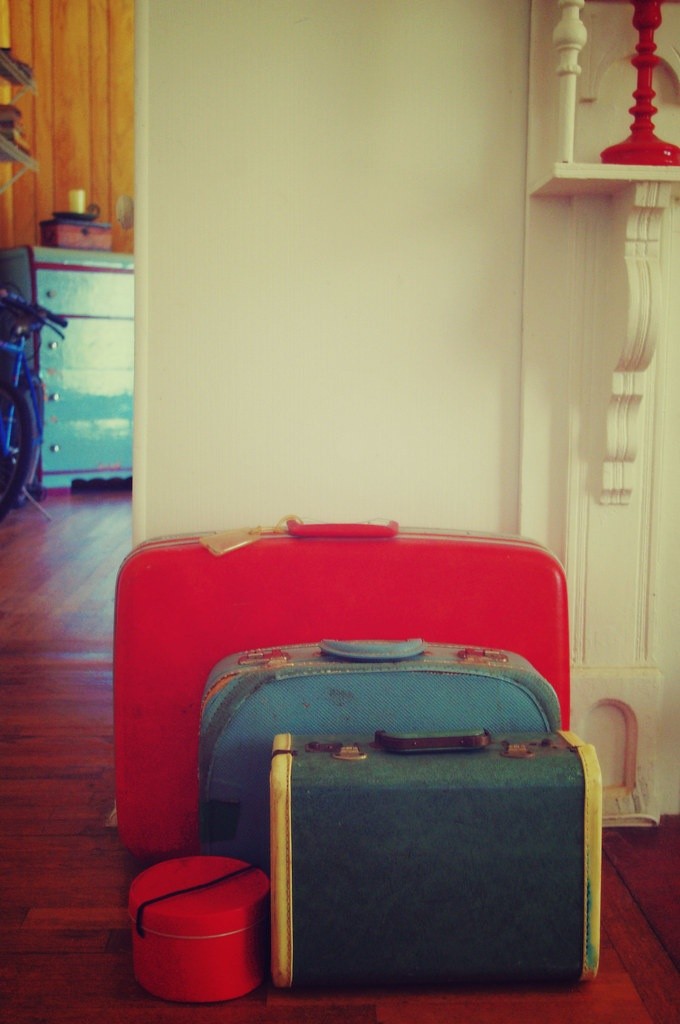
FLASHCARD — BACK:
[128,855,271,1004]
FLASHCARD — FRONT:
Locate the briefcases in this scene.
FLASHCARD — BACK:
[270,727,600,990]
[198,637,561,876]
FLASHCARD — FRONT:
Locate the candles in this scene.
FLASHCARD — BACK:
[68,189,86,214]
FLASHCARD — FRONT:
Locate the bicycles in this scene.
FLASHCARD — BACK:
[0,279,70,519]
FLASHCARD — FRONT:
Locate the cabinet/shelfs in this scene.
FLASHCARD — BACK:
[0,244,134,489]
[0,47,43,196]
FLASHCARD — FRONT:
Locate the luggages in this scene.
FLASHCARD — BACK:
[114,516,571,861]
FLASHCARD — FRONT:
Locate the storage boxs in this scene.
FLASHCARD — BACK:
[126,855,272,1002]
[39,219,113,251]
